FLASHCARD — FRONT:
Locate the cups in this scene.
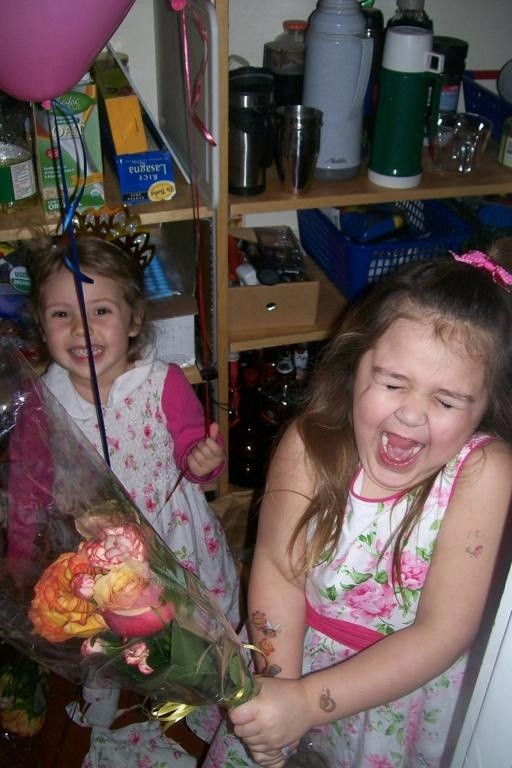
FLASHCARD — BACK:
[272,106,324,194]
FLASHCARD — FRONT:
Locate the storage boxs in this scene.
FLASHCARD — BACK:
[225,223,321,333]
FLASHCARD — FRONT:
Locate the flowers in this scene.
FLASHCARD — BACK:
[0,641,49,765]
[0,339,332,767]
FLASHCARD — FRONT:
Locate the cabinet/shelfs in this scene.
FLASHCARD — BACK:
[0,0,227,569]
[219,0,511,592]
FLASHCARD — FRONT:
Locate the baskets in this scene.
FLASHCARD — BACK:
[295,197,474,309]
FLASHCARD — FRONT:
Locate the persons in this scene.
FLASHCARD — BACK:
[186,249,512,768]
[0,231,241,768]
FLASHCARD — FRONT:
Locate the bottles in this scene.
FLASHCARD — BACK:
[227,68,276,196]
[359,2,511,177]
[229,342,316,488]
[262,19,307,104]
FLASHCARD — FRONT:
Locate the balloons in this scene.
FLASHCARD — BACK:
[0,0,137,110]
[171,0,186,11]
